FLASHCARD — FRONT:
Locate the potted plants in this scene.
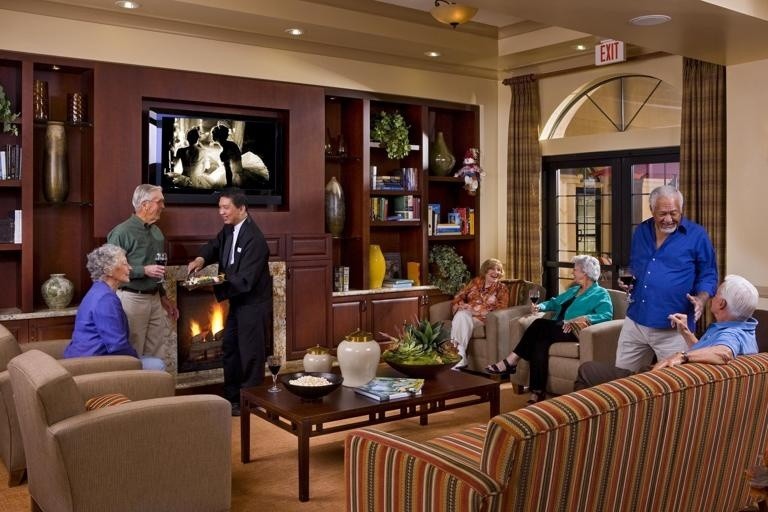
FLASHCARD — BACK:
[378,312,463,378]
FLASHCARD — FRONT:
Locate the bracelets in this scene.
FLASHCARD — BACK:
[679,349,687,362]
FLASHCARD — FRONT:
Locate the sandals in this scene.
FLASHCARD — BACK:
[523,391,545,408]
[484,359,516,375]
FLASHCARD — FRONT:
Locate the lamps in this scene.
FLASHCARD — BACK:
[430,0,478,28]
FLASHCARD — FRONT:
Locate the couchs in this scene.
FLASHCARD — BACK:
[344,350,768,512]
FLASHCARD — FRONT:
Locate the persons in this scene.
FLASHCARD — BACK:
[61,243,168,372]
[448,258,509,367]
[486,253,613,404]
[103,182,180,367]
[173,125,209,187]
[614,184,718,379]
[212,124,269,189]
[572,272,759,394]
[186,185,275,420]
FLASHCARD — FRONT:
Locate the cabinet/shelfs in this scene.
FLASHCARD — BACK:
[0,48,94,341]
[266,233,332,360]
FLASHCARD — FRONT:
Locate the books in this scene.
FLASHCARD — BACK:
[0,218,15,244]
[0,143,24,182]
[426,203,475,235]
[360,376,425,394]
[382,252,402,276]
[382,278,414,289]
[406,261,421,287]
[371,165,422,224]
[353,377,423,400]
[14,209,22,243]
[334,265,350,293]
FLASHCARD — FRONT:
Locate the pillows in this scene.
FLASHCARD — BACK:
[85,391,133,411]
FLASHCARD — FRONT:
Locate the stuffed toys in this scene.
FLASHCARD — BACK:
[453,148,486,196]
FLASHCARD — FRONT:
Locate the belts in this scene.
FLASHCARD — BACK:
[118,287,158,295]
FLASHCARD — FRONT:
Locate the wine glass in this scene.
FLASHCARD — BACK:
[618,266,637,305]
[265,355,282,393]
[155,252,171,285]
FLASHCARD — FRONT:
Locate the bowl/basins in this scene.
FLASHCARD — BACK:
[281,372,344,402]
[382,350,463,382]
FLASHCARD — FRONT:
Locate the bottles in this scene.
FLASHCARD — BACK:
[42,273,74,311]
[369,245,387,289]
[429,132,456,175]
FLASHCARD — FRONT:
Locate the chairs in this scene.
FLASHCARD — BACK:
[7,348,232,512]
[430,279,547,383]
[0,324,143,487]
[507,289,629,395]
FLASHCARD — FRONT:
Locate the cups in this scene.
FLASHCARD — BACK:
[529,290,541,304]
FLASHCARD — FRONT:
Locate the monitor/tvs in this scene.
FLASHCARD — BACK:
[149,107,283,204]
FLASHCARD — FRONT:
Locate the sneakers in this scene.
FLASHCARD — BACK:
[455,357,467,369]
[232,404,255,416]
